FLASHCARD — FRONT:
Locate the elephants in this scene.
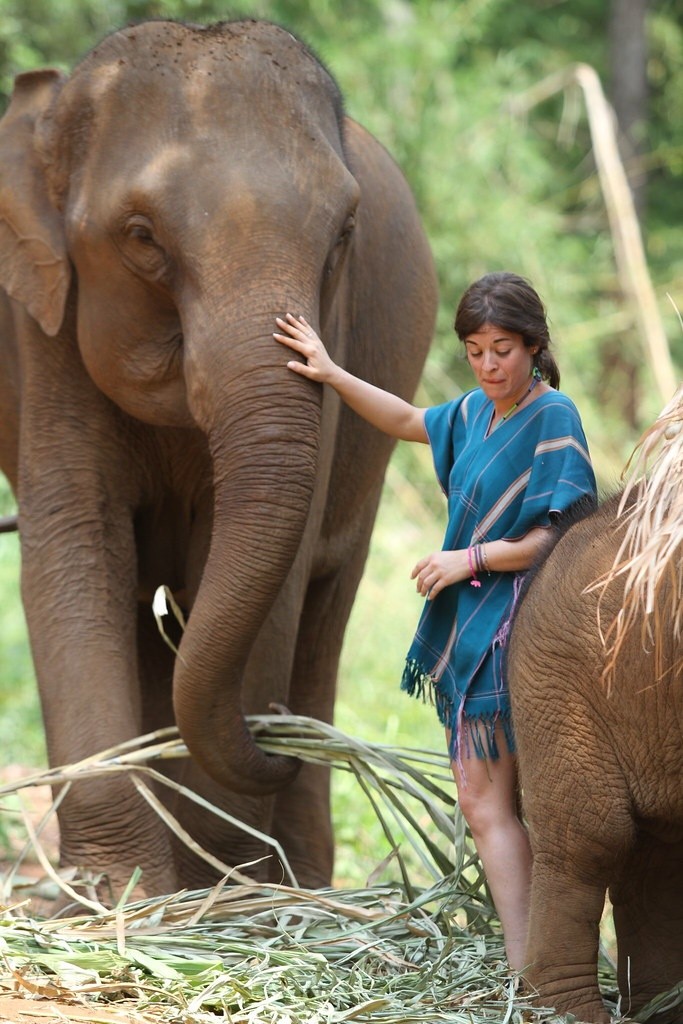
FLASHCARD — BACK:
[1,13,441,922]
[506,475,683,1023]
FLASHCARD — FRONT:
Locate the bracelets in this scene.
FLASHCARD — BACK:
[467,544,492,589]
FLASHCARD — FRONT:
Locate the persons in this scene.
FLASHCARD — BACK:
[272,272,598,982]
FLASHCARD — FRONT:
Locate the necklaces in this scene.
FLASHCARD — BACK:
[493,366,543,432]
